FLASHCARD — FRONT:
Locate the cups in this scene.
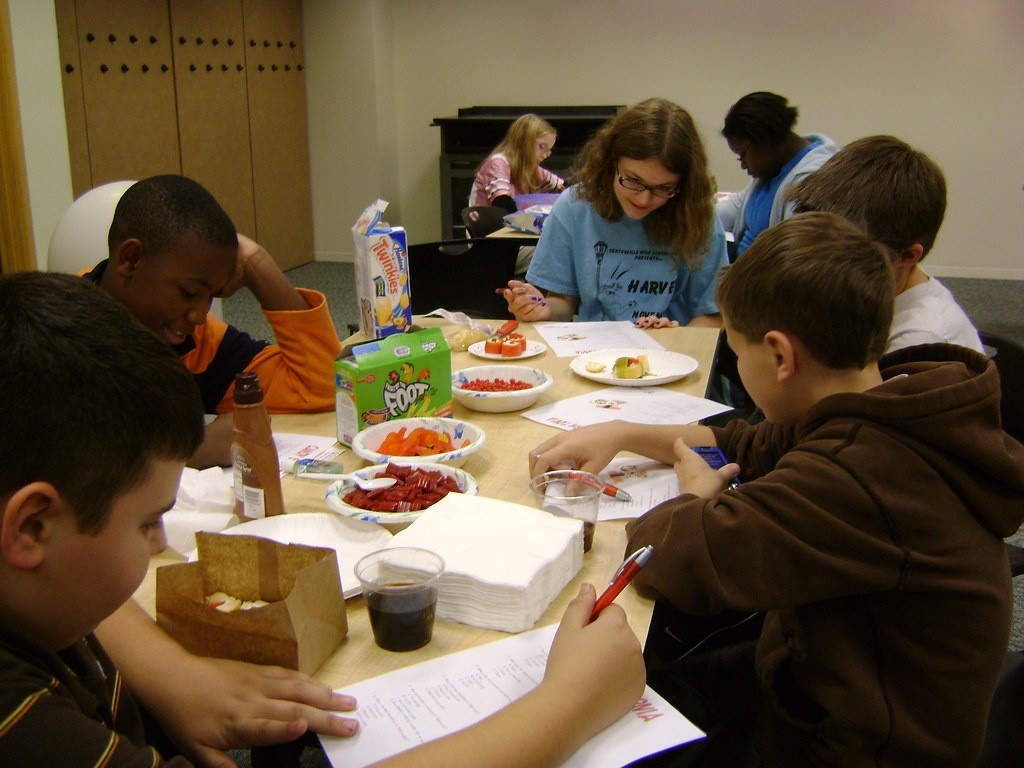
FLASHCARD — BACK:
[530,470,606,555]
[353,546,444,651]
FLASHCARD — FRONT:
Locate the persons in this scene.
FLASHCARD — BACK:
[527,212,1024,768]
[504,97,731,329]
[63,175,346,469]
[711,91,844,262]
[739,135,985,485]
[466,113,569,274]
[0,271,647,768]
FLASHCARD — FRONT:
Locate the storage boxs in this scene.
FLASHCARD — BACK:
[333,324,453,447]
[513,193,560,212]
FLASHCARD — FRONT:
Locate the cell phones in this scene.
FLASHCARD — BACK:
[692,445,740,492]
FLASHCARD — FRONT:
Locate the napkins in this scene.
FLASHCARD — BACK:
[376,490,588,636]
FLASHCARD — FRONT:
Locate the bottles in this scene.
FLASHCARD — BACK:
[231,371,285,520]
[282,456,342,473]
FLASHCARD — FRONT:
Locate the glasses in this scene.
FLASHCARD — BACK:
[614,160,681,199]
[736,137,758,168]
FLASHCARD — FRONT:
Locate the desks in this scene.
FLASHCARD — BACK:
[486,224,538,250]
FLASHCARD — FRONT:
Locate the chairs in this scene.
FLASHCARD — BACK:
[407,204,522,322]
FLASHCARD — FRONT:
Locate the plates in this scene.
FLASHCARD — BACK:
[468,339,546,361]
[570,347,700,386]
[190,512,394,601]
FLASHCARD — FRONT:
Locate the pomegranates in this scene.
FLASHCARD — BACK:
[460,378,533,391]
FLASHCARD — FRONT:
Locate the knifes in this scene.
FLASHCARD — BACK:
[490,319,518,341]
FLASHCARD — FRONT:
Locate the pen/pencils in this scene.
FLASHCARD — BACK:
[559,185,566,191]
[495,288,526,294]
[590,544,655,625]
[546,468,631,502]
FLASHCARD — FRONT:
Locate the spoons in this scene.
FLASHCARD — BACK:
[298,473,398,491]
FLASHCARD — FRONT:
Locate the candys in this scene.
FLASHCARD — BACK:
[378,426,471,456]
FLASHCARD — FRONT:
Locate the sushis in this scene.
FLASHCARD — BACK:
[485,334,527,357]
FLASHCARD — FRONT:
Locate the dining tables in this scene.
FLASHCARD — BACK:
[125,313,720,694]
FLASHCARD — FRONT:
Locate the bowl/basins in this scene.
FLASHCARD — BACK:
[351,417,484,468]
[452,365,554,412]
[325,463,478,534]
